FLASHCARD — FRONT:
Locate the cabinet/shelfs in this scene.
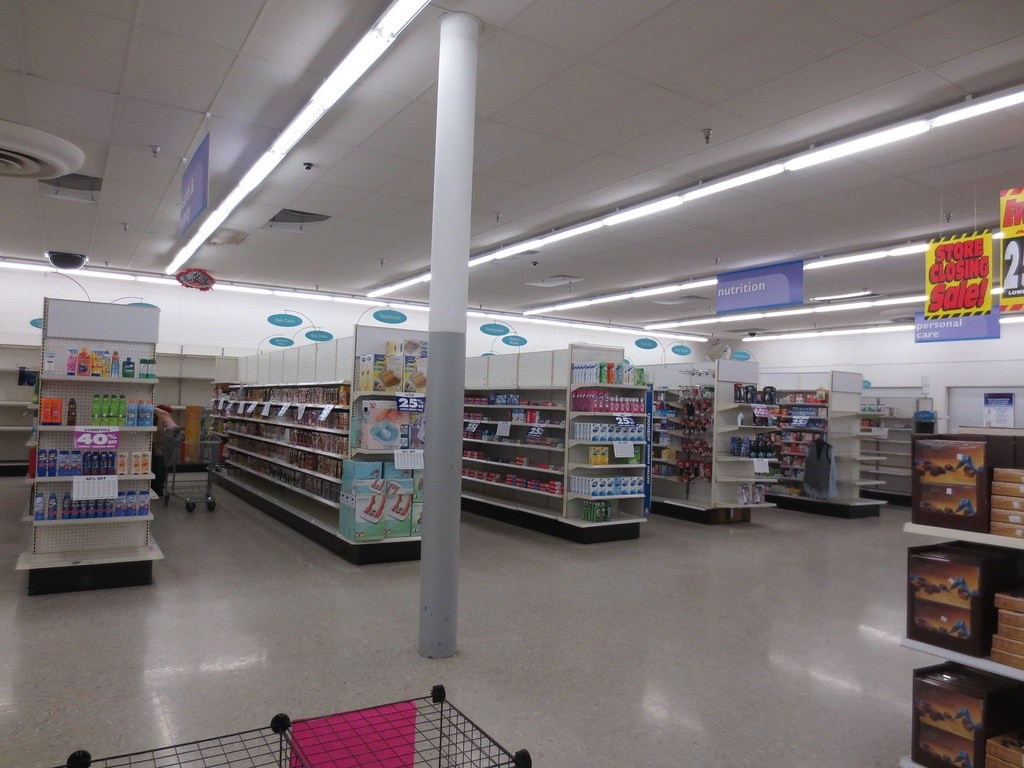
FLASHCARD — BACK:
[209,380,350,510]
[0,340,214,470]
[464,379,648,532]
[351,391,427,457]
[899,425,1024,768]
[652,375,913,510]
[20,370,160,526]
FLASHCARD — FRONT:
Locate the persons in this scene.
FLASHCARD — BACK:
[151,404,180,498]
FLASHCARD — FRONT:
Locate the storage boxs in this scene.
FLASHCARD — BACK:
[570,421,647,499]
[906,433,1024,767]
[337,339,428,541]
[182,405,202,464]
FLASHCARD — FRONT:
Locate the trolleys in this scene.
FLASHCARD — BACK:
[53,685,532,768]
[161,425,223,514]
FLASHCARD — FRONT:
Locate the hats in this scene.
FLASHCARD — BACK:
[157,404,173,414]
